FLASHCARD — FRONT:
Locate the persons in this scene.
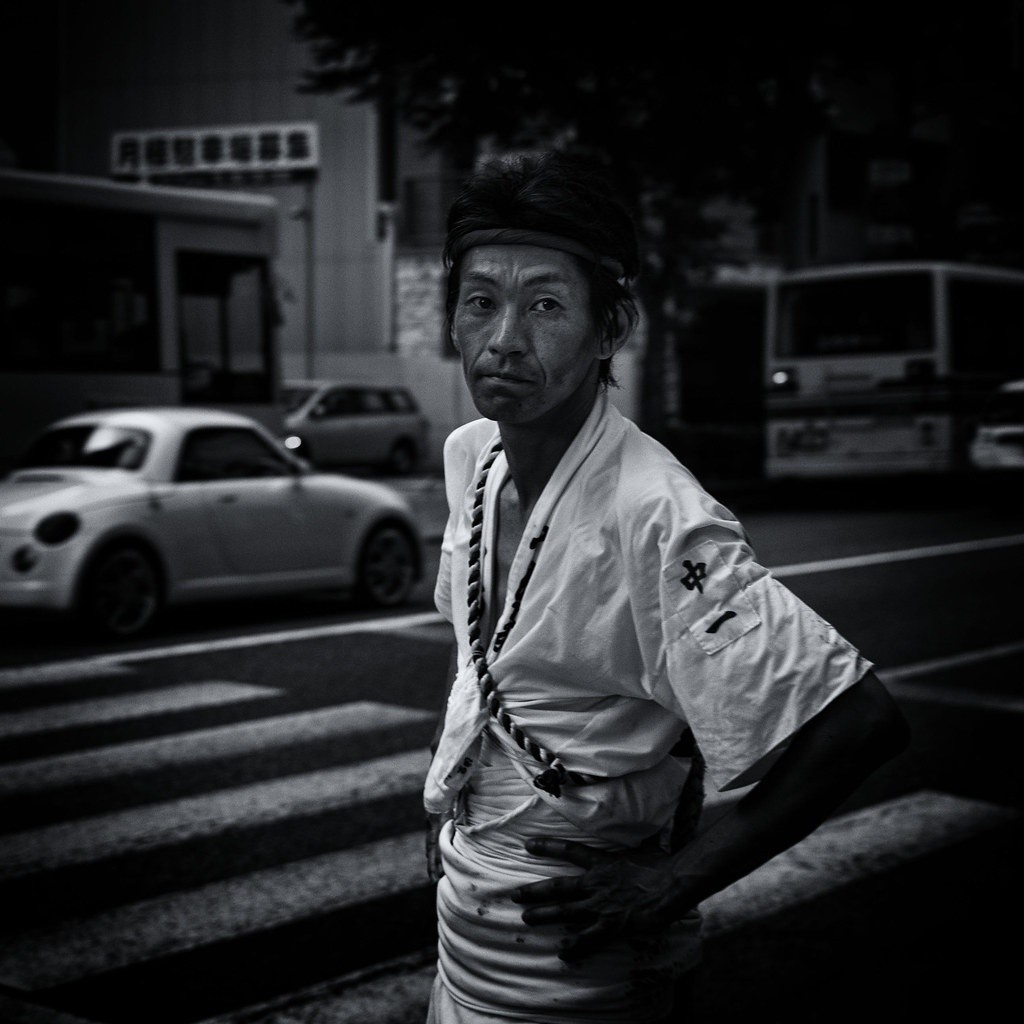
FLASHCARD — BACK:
[420,148,911,1024]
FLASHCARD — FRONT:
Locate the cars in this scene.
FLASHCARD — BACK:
[284,375,427,474]
[1,403,428,642]
[969,380,1024,476]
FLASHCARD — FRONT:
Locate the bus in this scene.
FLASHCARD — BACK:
[762,262,1024,485]
